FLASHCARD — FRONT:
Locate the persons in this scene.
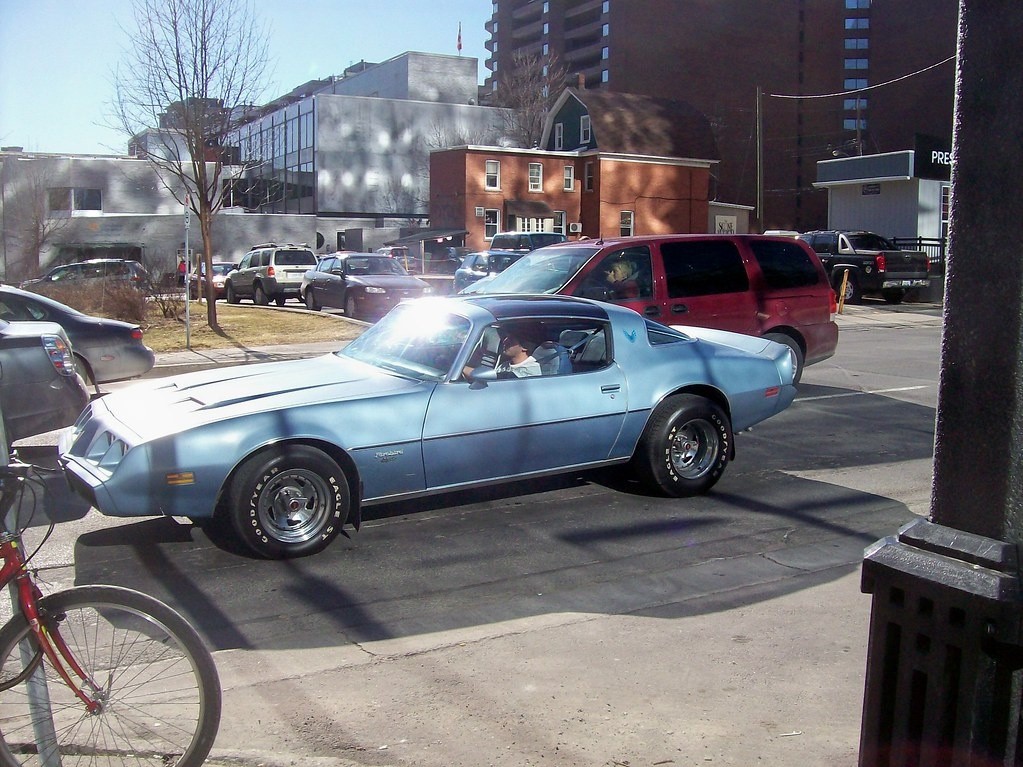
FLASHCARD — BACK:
[604,260,642,299]
[462,326,542,383]
[176,261,186,287]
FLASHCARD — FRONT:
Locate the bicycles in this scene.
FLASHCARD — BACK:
[1,410,222,767]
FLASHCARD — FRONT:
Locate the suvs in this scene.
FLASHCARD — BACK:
[223,243,320,303]
[19,259,152,288]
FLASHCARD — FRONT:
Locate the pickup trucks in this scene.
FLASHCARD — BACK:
[796,229,931,306]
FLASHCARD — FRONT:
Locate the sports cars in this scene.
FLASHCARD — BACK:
[55,290,800,562]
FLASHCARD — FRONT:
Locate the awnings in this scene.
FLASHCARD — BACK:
[506,199,558,219]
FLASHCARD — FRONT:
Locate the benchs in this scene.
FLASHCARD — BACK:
[560,329,608,371]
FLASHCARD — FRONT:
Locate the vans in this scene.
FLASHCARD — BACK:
[479,231,842,390]
[487,231,570,276]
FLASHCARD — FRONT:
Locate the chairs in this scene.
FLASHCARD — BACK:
[532,341,575,375]
[480,327,503,370]
[367,260,385,272]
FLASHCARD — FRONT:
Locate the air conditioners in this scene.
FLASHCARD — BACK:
[569,222,582,232]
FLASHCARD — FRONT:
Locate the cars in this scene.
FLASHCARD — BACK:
[0,281,158,385]
[454,251,526,293]
[0,319,93,441]
[189,261,238,304]
[299,251,438,318]
